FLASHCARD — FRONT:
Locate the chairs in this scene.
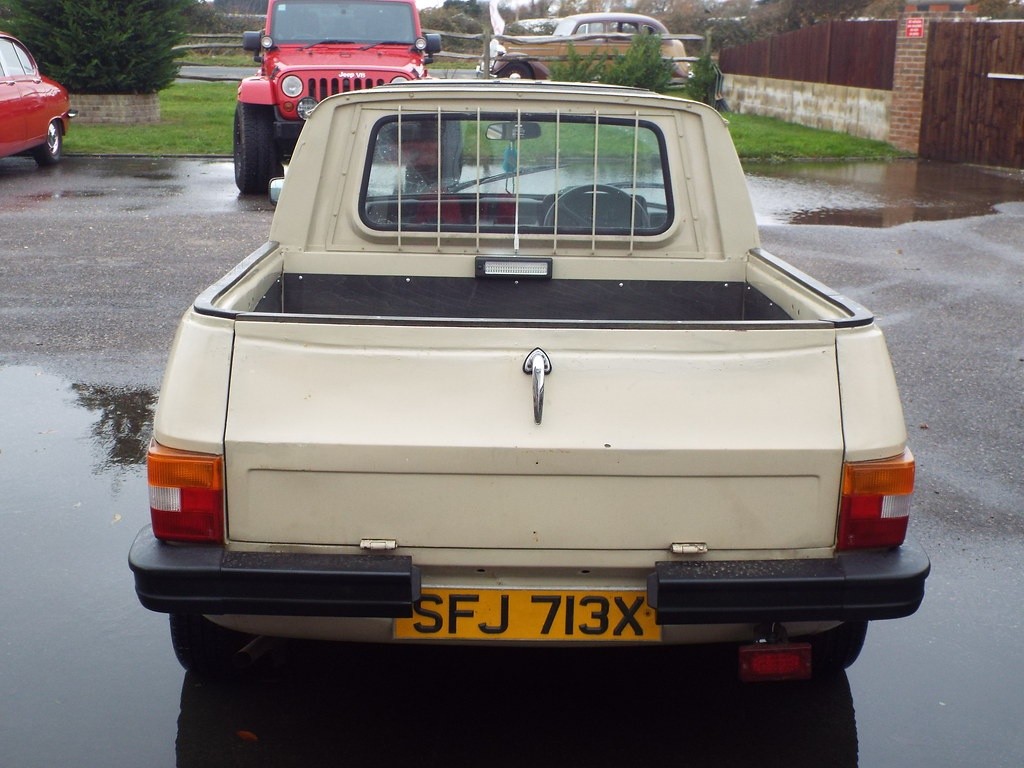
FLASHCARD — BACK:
[540,183,649,230]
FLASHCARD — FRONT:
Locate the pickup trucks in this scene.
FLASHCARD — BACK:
[126,78,932,685]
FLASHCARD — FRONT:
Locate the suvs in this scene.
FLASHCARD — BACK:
[232,0,463,195]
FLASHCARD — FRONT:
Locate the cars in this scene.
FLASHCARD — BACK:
[475,12,691,92]
[0,33,72,163]
